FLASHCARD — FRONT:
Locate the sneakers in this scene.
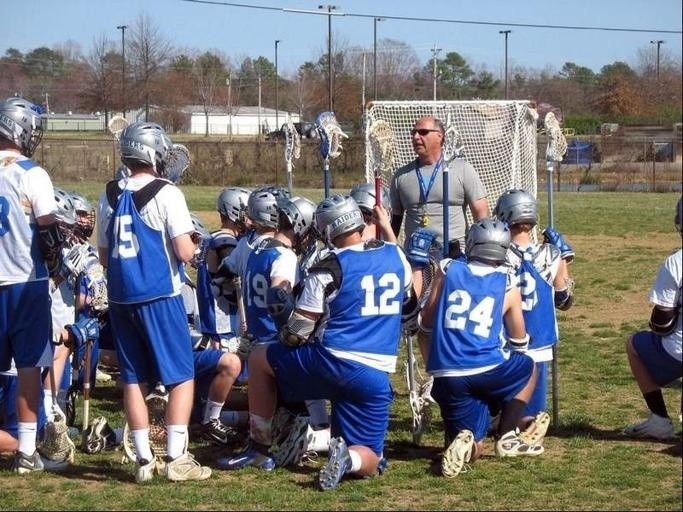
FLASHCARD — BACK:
[167,450,212,482]
[271,406,292,444]
[318,436,352,491]
[517,411,551,447]
[135,454,166,482]
[84,416,116,455]
[66,426,79,439]
[495,430,544,458]
[214,436,276,472]
[276,416,317,469]
[201,418,238,445]
[626,413,674,441]
[16,449,74,475]
[441,429,474,479]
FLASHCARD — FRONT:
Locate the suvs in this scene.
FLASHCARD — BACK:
[266,122,317,140]
[560,140,603,163]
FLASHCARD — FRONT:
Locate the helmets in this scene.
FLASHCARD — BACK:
[465,218,512,266]
[280,196,322,256]
[351,184,393,227]
[217,186,255,234]
[67,191,96,237]
[497,189,537,230]
[316,194,366,250]
[54,188,78,241]
[189,213,213,270]
[120,121,173,178]
[248,185,291,231]
[1,97,45,158]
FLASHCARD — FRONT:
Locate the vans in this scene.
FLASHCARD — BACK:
[539,128,576,137]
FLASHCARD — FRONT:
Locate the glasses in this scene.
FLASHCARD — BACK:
[411,129,439,135]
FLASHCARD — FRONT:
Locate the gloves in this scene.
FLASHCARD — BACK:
[405,229,438,267]
[543,227,575,265]
[48,257,62,279]
[65,317,100,352]
[220,336,256,361]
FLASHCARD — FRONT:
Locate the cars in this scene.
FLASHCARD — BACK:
[638,141,676,162]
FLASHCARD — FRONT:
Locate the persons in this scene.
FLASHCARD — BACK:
[390,116,491,405]
[491,189,574,450]
[177,218,250,447]
[240,196,331,469]
[213,193,413,492]
[196,185,255,362]
[69,193,108,400]
[349,182,394,241]
[0,189,76,457]
[623,193,683,441]
[204,187,292,471]
[416,218,546,482]
[0,97,65,478]
[95,121,212,487]
